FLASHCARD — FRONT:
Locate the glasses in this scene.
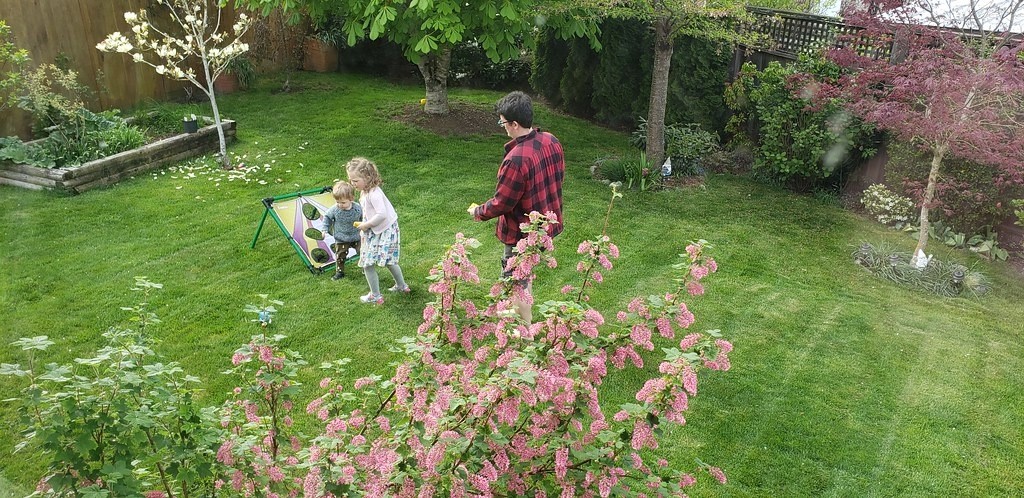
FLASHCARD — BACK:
[498,119,508,128]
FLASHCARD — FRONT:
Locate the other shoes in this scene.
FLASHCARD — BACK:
[331,273,344,281]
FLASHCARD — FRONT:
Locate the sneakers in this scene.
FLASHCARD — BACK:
[360,292,384,305]
[388,284,410,292]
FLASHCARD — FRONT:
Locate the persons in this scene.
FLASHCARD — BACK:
[466,90,565,341]
[345,158,411,304]
[322,179,366,280]
